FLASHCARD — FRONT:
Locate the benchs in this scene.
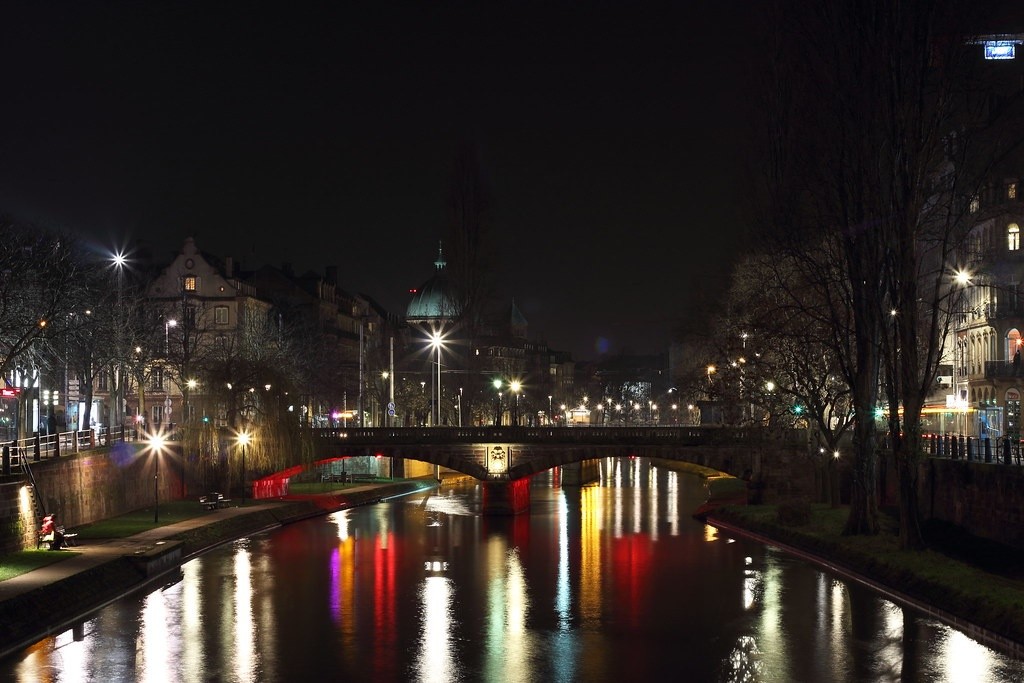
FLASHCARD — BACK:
[199,492,232,512]
[322,474,376,484]
[37,525,78,550]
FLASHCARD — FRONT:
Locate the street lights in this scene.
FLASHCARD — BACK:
[238,433,248,506]
[433,336,441,426]
[151,437,161,522]
[62,310,93,432]
[165,319,177,427]
[493,380,501,428]
[512,381,519,426]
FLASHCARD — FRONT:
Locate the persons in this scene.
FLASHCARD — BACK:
[348,419,356,428]
[73,411,77,423]
[42,513,68,548]
